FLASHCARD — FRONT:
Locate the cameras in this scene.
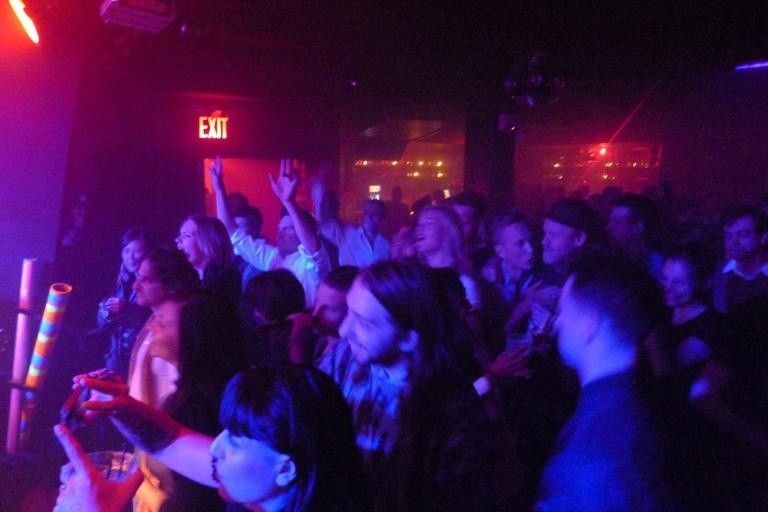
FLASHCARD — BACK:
[53,383,93,447]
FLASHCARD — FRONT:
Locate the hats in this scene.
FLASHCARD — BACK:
[543,197,608,242]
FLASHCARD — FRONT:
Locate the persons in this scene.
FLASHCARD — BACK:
[48,153,768,511]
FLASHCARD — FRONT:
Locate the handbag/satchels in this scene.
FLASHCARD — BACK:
[39,382,120,485]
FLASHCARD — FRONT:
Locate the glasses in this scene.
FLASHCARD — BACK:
[655,266,703,285]
